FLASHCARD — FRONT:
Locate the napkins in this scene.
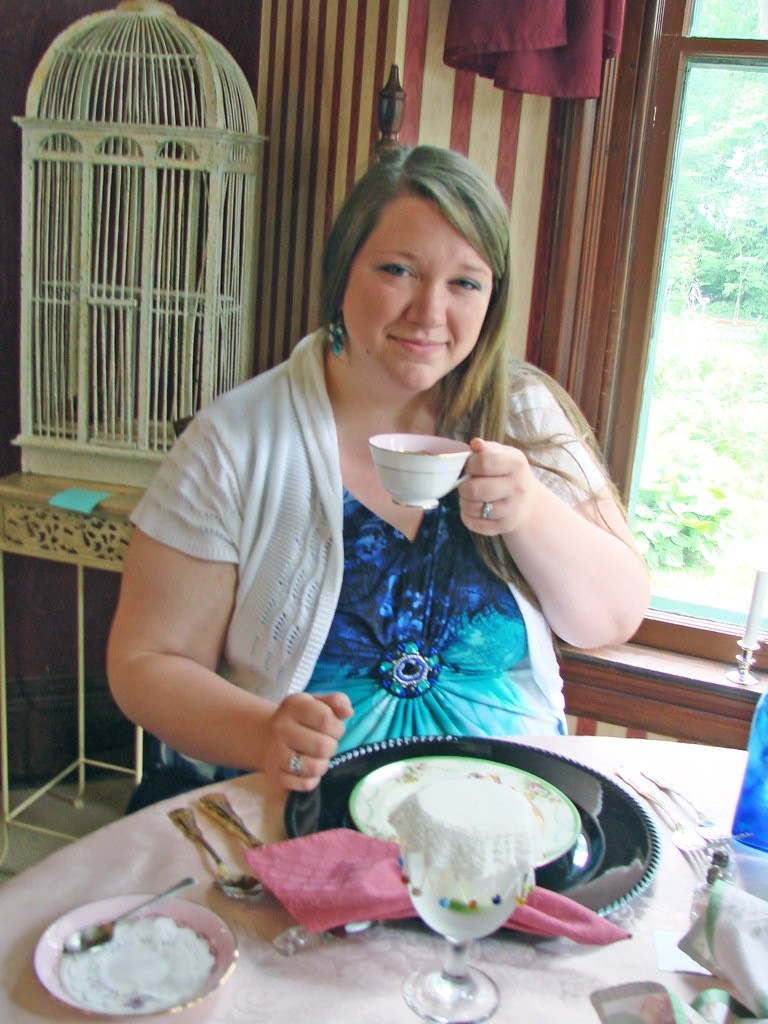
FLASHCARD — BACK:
[245,828,633,946]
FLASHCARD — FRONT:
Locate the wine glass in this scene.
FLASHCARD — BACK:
[402,780,535,1024]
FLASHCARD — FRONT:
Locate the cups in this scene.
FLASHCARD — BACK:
[367,432,473,511]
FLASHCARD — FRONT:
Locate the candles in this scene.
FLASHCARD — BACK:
[742,569,768,647]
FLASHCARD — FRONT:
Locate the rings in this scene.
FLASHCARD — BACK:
[480,502,492,520]
[289,752,303,776]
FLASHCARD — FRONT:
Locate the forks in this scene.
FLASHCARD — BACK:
[641,768,754,844]
[615,769,713,882]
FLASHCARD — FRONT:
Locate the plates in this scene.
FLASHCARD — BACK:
[348,755,581,872]
[32,894,239,1019]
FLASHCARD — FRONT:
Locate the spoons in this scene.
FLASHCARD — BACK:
[167,807,269,902]
[62,875,197,956]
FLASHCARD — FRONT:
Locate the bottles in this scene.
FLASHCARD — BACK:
[731,689,768,854]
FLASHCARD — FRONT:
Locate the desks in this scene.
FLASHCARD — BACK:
[0,735,748,1024]
[0,473,144,841]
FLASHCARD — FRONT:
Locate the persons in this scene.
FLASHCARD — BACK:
[105,145,645,816]
